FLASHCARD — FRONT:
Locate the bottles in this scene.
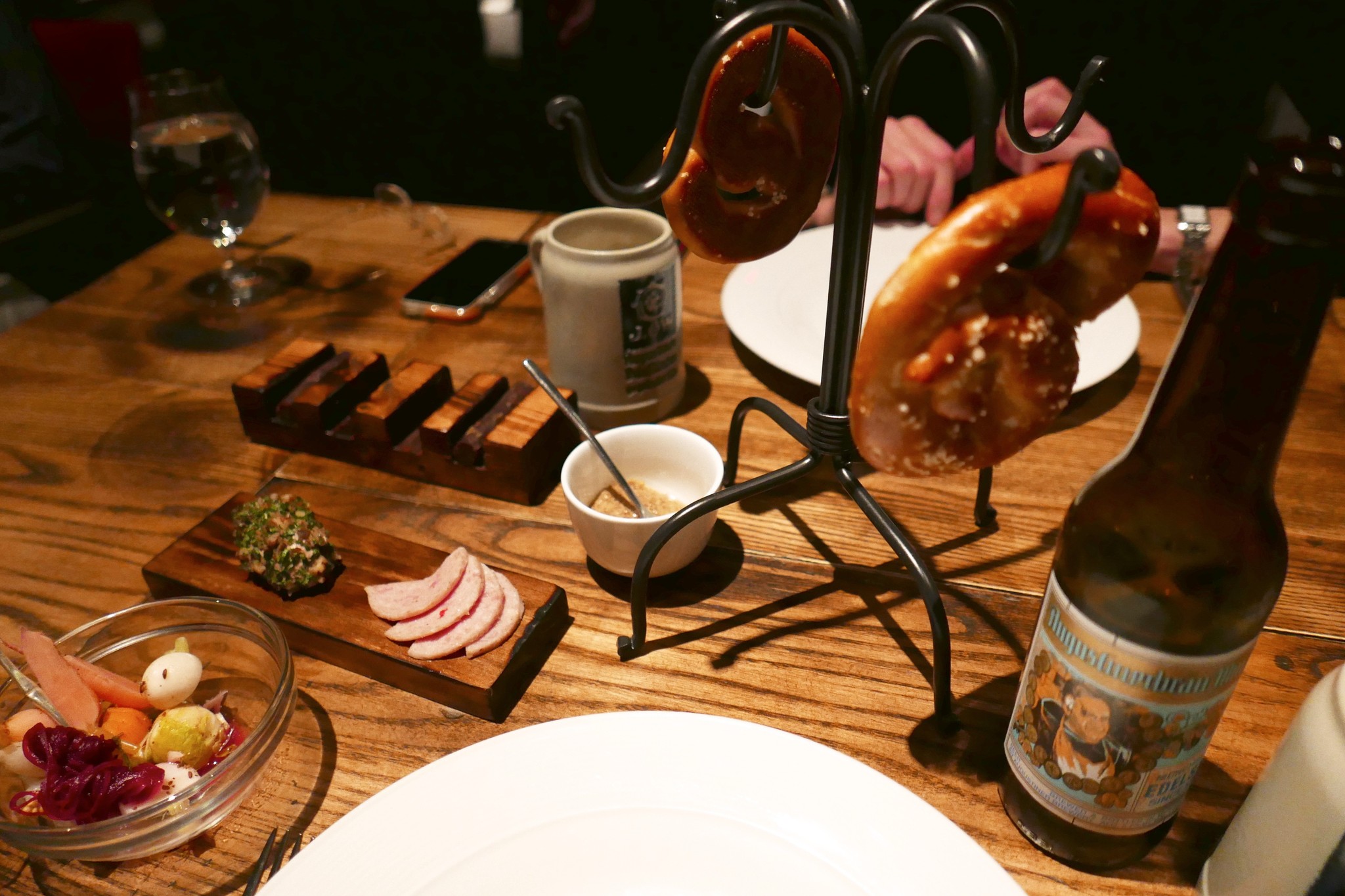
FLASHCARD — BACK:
[1198,662,1344,896]
[999,129,1345,870]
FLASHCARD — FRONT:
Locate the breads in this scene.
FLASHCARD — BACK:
[849,157,1165,477]
[660,28,843,264]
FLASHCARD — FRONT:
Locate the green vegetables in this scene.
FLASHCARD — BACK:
[234,497,335,592]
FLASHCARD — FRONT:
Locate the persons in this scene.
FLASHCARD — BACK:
[0,0,1345,332]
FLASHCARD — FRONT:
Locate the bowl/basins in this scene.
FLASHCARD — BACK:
[0,601,293,860]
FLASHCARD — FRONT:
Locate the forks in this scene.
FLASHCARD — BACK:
[241,826,315,896]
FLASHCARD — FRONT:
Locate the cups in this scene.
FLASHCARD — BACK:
[527,206,685,433]
[561,422,726,577]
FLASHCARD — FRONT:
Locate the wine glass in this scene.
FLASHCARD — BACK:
[129,70,308,308]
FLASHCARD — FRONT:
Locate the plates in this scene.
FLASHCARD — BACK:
[721,218,1139,394]
[257,710,1028,896]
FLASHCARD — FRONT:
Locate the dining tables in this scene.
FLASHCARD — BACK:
[0,193,1345,896]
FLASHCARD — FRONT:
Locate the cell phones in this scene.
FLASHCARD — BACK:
[400,238,533,320]
